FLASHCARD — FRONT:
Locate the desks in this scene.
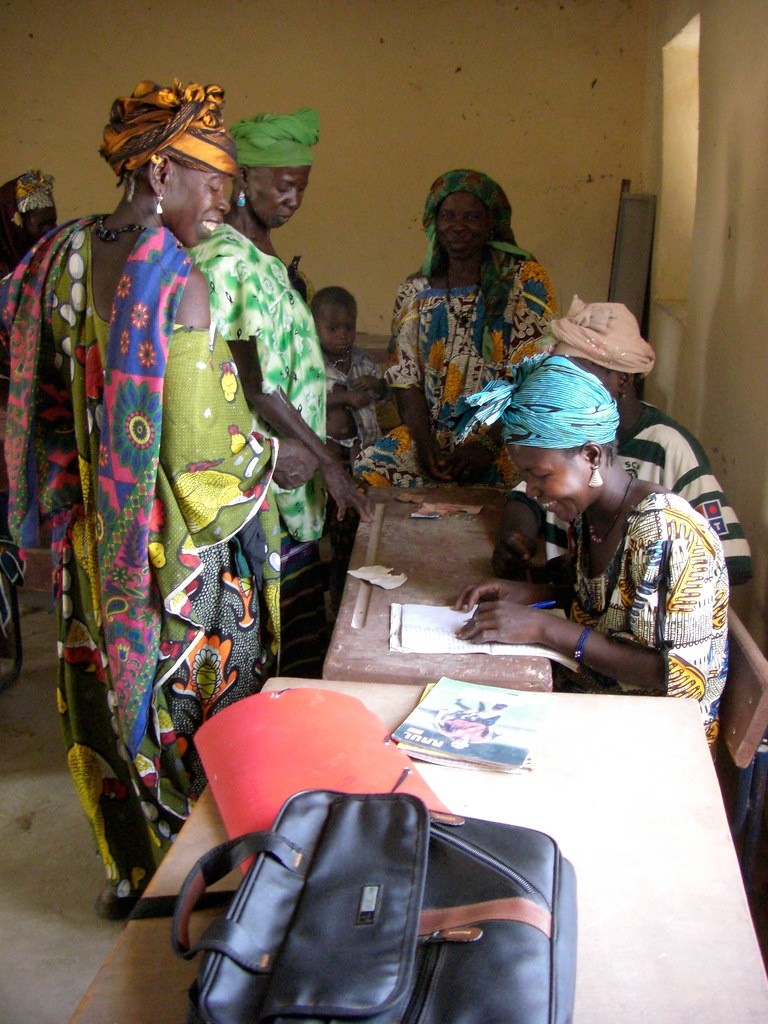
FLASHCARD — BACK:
[64,670,768,1024]
[319,485,554,696]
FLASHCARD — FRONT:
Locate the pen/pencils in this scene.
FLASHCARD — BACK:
[461,600,556,624]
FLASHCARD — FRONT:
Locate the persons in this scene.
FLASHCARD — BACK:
[0,76,394,918]
[380,168,753,769]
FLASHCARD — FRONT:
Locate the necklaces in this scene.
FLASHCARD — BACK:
[589,474,633,544]
[94,213,147,242]
[445,264,482,331]
[331,351,351,368]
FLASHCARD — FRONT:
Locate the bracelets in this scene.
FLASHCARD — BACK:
[574,626,592,664]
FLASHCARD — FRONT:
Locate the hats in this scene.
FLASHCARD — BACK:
[452,351,620,450]
[97,76,238,187]
[227,105,320,168]
[549,294,656,380]
[422,169,512,278]
[16,168,56,214]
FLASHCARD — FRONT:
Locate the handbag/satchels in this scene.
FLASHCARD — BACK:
[171,788,577,1024]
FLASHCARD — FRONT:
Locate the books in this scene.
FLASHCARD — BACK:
[389,602,581,674]
[389,676,550,775]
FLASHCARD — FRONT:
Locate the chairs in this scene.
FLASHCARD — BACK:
[708,607,768,836]
